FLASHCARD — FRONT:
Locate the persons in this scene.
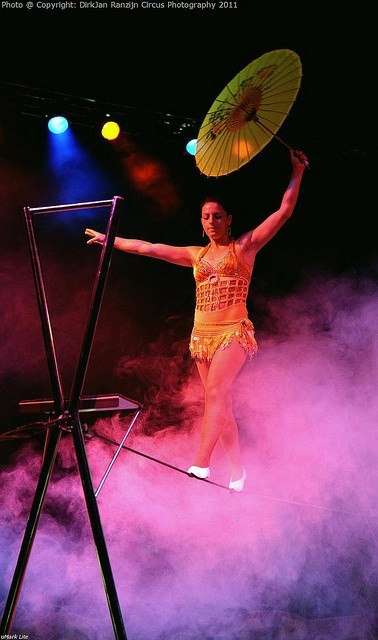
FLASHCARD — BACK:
[84,149,308,492]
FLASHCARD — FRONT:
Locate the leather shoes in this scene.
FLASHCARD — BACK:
[228,467,246,497]
[187,466,210,479]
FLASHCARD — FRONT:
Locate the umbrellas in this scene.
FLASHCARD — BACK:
[195,48,303,180]
